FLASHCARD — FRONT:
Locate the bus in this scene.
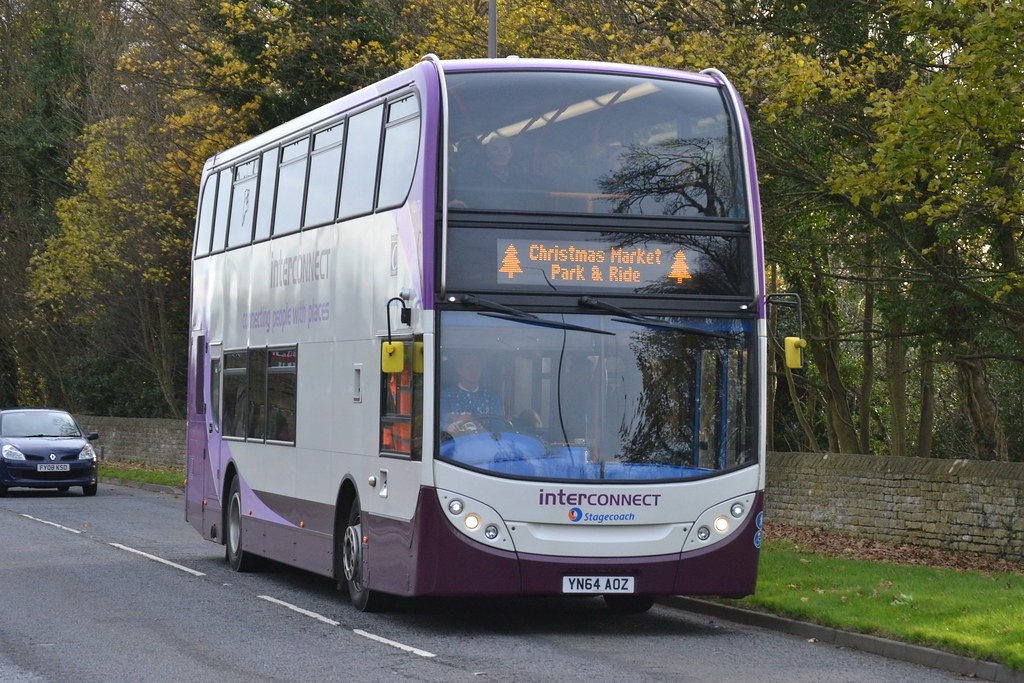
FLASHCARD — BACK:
[187,51,810,620]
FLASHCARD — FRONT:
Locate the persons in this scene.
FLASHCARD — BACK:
[438,350,506,436]
[473,134,536,188]
[518,408,542,429]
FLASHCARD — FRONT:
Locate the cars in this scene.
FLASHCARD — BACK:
[1,408,101,497]
